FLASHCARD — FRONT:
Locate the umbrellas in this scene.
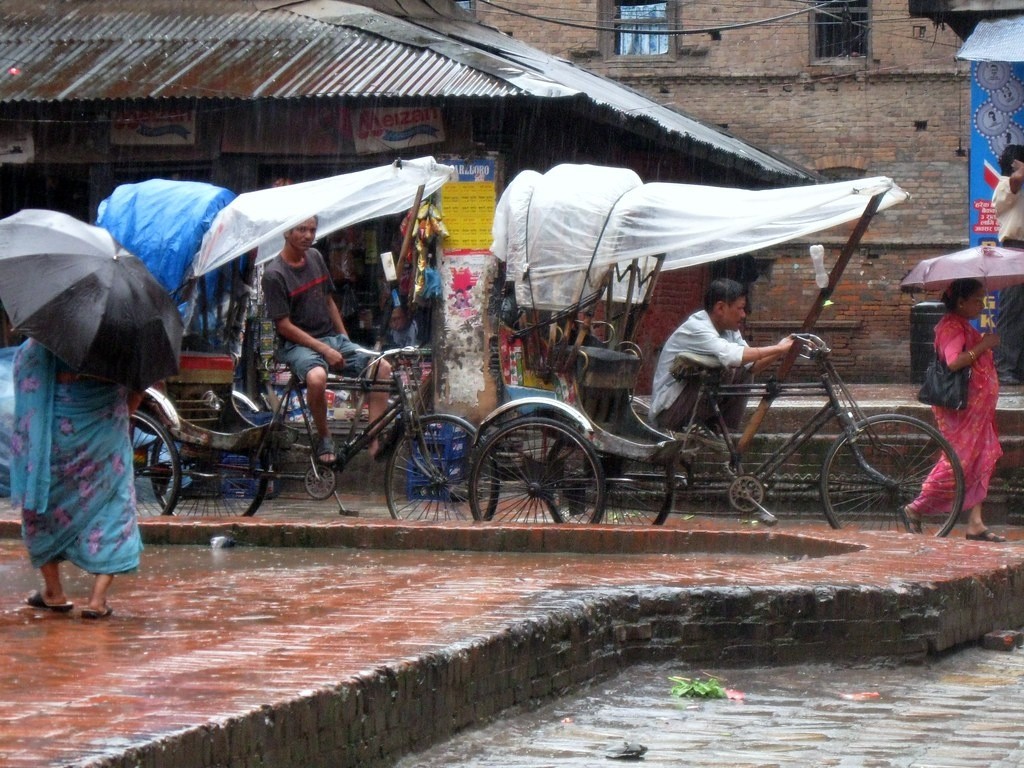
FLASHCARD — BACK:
[900,246,1024,335]
[0,209,184,394]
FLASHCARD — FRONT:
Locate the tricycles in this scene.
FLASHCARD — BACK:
[470,176,965,542]
[129,156,499,528]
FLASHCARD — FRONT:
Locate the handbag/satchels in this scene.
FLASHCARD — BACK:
[917,321,972,410]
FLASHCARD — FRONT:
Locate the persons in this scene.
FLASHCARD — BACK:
[649,278,796,450]
[261,215,391,464]
[389,301,421,347]
[993,144,1024,385]
[897,278,1006,543]
[9,336,144,618]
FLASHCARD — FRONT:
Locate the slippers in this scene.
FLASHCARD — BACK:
[898,505,922,533]
[315,437,340,465]
[374,422,406,462]
[24,591,74,612]
[966,529,1007,542]
[82,605,112,618]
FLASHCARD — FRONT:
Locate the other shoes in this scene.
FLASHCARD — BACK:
[674,418,728,452]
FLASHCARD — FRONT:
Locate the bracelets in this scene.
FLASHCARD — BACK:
[968,349,976,360]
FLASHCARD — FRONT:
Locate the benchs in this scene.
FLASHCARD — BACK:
[498,297,645,389]
[163,331,233,386]
